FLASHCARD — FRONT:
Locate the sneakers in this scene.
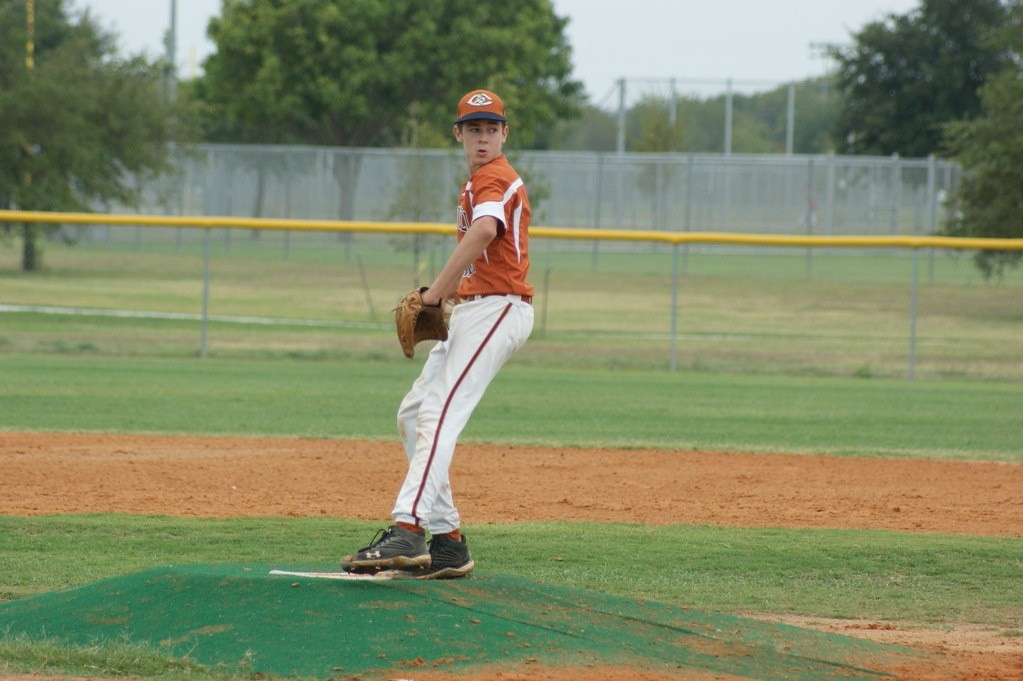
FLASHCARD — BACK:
[338,526,432,575]
[391,534,476,580]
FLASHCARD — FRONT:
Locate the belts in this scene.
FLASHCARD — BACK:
[466,293,532,304]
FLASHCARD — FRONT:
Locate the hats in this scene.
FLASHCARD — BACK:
[454,90,506,124]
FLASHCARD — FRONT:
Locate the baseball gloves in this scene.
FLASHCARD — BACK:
[394,285,450,362]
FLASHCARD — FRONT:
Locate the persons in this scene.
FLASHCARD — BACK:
[341,89,535,580]
